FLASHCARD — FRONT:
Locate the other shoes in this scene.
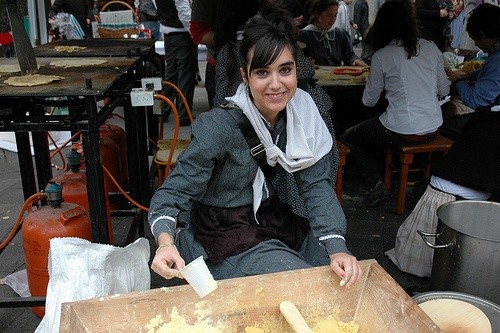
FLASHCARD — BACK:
[162,114,169,122]
[179,117,196,126]
[364,182,388,207]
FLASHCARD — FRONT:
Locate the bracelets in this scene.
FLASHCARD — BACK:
[156,243,177,255]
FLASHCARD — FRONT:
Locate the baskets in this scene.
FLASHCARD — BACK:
[97,0,141,39]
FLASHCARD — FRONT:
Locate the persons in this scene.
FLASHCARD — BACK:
[49,0,94,39]
[437,3,500,145]
[343,0,451,207]
[135,0,161,41]
[458,0,500,61]
[157,0,197,127]
[190,0,339,220]
[148,19,362,288]
[283,0,369,67]
[415,0,455,53]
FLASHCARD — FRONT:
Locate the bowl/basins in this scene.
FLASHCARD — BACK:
[412,291,500,333]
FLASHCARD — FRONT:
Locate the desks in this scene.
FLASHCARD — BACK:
[312,62,461,140]
[57,259,446,333]
[0,35,165,249]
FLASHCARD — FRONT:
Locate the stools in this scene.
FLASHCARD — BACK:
[332,139,351,208]
[383,134,454,217]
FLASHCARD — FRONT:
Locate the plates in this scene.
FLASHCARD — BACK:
[333,68,362,75]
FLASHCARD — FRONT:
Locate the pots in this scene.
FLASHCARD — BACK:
[417,200,500,312]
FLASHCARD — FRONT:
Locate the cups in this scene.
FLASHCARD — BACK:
[179,255,218,298]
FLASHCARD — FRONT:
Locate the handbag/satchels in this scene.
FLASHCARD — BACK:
[189,99,312,261]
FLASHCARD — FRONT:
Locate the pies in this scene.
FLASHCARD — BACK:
[53,46,87,52]
[49,59,107,68]
[3,74,66,86]
